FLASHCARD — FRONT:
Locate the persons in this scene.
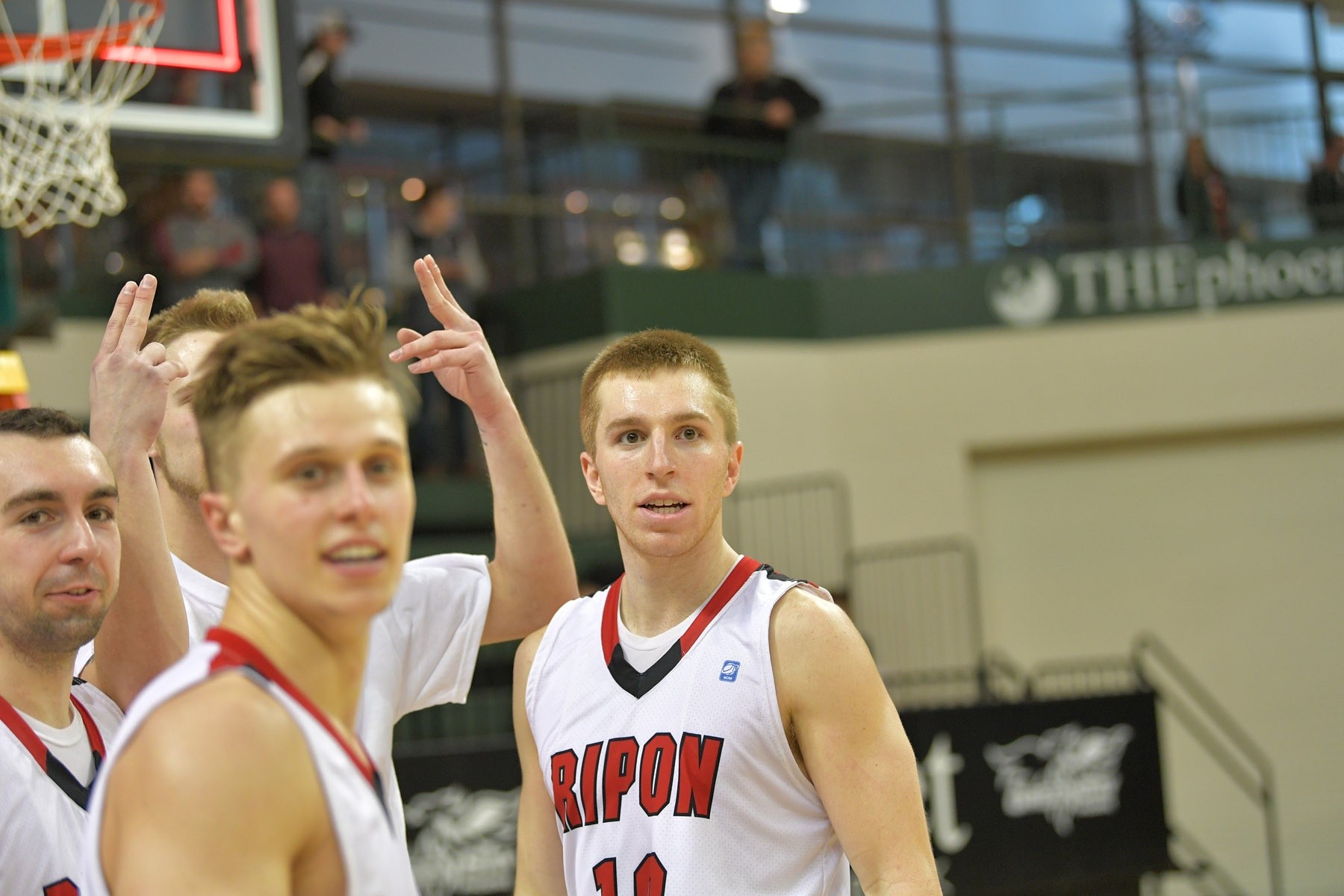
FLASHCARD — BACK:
[85,307,417,896]
[0,408,126,896]
[1303,128,1344,243]
[74,253,579,896]
[0,15,489,349]
[1174,132,1233,240]
[513,329,945,896]
[697,21,822,270]
